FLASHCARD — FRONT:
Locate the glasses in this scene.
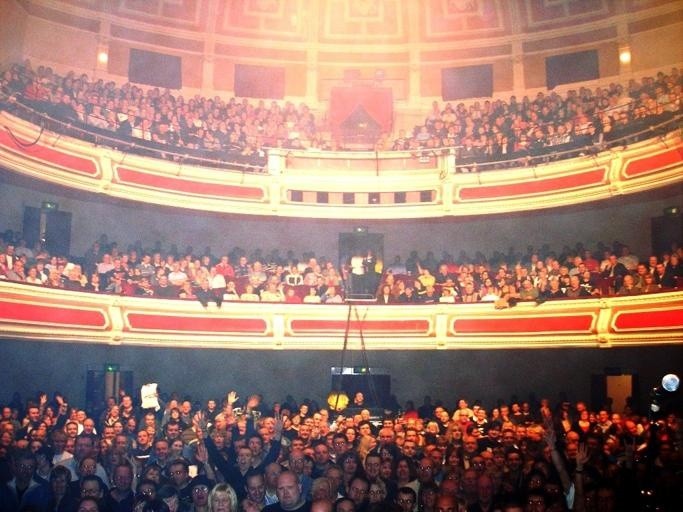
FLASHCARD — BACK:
[19,439,640,505]
